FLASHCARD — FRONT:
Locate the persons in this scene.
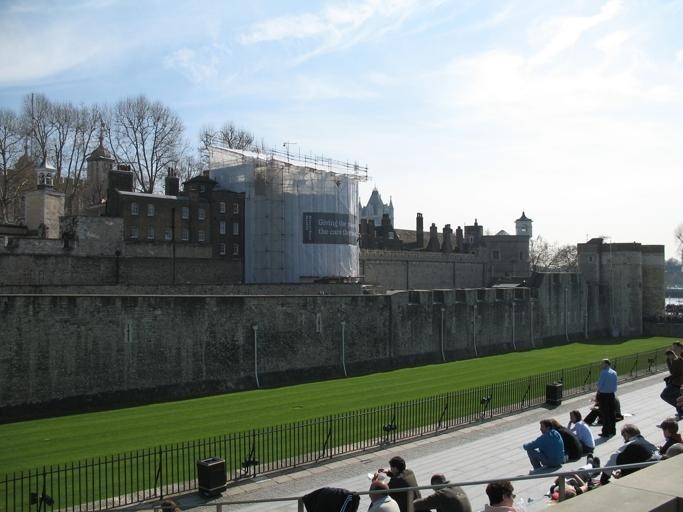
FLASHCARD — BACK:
[566,411,597,453]
[414,474,472,512]
[550,420,582,462]
[522,419,566,474]
[673,342,683,361]
[367,456,421,512]
[599,424,663,485]
[584,391,620,425]
[550,451,599,499]
[660,350,683,420]
[593,359,618,437]
[368,482,401,512]
[482,479,521,512]
[657,420,682,455]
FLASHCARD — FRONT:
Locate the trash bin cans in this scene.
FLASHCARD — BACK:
[198,457,227,498]
[546,382,563,406]
